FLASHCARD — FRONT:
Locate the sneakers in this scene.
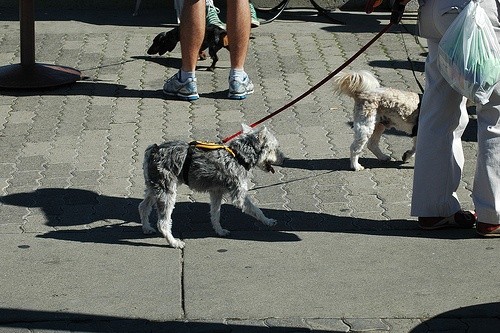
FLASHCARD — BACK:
[205,0,226,31]
[475,221,500,238]
[227,71,255,100]
[249,3,260,27]
[417,209,477,231]
[162,69,200,101]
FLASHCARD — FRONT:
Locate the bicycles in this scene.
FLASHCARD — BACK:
[248,0,344,25]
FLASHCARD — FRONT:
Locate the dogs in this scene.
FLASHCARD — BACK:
[334,69,424,171]
[138,123,287,249]
[147,22,230,72]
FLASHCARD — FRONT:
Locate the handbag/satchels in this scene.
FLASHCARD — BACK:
[436,0,500,106]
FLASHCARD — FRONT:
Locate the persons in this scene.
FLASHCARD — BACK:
[162,0,260,100]
[411,0,500,237]
